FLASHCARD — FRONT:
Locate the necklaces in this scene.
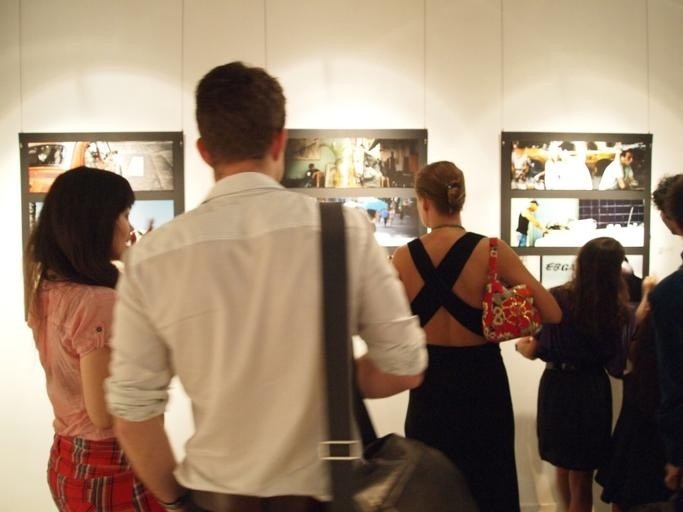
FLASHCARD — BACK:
[431,224,464,233]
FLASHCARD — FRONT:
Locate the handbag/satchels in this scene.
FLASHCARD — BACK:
[319,432,476,512]
[481,235,543,344]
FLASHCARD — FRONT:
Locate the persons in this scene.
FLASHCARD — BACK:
[23,167,161,511]
[515,237,656,510]
[392,162,564,511]
[102,61,430,511]
[301,152,406,228]
[510,141,639,191]
[593,252,682,511]
[514,199,548,247]
[651,174,682,236]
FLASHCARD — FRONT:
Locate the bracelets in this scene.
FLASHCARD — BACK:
[162,496,184,508]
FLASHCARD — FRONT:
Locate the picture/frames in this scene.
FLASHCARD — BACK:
[18,131,184,246]
[500,131,653,289]
[281,128,429,254]
